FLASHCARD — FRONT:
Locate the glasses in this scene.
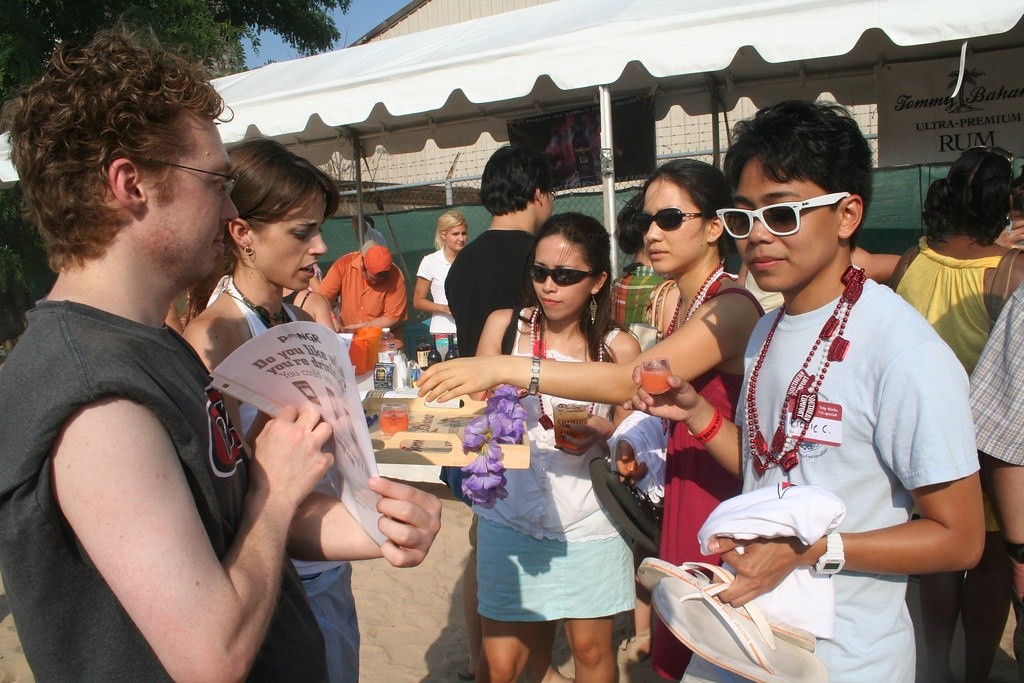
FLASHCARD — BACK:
[716,191,852,239]
[960,144,1015,191]
[136,155,241,197]
[630,207,713,234]
[529,263,597,286]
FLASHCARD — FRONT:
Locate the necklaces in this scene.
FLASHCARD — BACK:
[747,265,867,477]
[233,280,287,327]
[661,262,724,435]
[531,301,603,430]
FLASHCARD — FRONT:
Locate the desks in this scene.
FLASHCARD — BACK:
[357,371,458,485]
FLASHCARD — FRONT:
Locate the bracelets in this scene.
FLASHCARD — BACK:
[688,408,725,446]
[529,357,542,398]
[1002,539,1024,565]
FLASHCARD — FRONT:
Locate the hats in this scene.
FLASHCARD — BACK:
[361,240,393,279]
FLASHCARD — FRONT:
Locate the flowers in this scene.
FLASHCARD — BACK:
[461,384,528,508]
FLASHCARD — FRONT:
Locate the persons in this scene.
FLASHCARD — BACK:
[179,138,359,683]
[623,101,984,683]
[0,18,444,683]
[416,146,784,683]
[465,212,641,683]
[165,210,467,376]
[851,145,1024,683]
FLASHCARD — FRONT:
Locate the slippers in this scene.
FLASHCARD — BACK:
[637,557,826,683]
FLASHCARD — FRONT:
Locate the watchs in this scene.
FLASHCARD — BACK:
[808,531,845,575]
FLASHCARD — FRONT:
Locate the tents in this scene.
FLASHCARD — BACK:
[0,1,1024,274]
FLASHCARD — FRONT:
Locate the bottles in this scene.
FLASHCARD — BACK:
[378,328,397,364]
[428,335,442,368]
[416,336,433,371]
[389,342,400,364]
[445,334,458,360]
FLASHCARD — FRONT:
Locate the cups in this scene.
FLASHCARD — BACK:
[550,396,594,451]
[640,358,672,395]
[379,403,410,437]
